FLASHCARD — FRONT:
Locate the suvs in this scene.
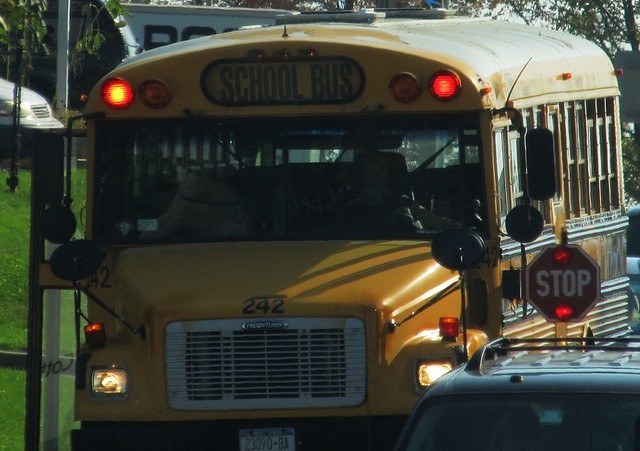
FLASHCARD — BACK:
[391,334,640,450]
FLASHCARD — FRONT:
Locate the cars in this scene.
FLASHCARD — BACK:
[1,78,64,159]
[625,205,639,296]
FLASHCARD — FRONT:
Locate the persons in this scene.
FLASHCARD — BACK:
[322,148,413,212]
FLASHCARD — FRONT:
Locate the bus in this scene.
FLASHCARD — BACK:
[49,8,631,451]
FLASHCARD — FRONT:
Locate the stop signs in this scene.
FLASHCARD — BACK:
[525,244,601,323]
